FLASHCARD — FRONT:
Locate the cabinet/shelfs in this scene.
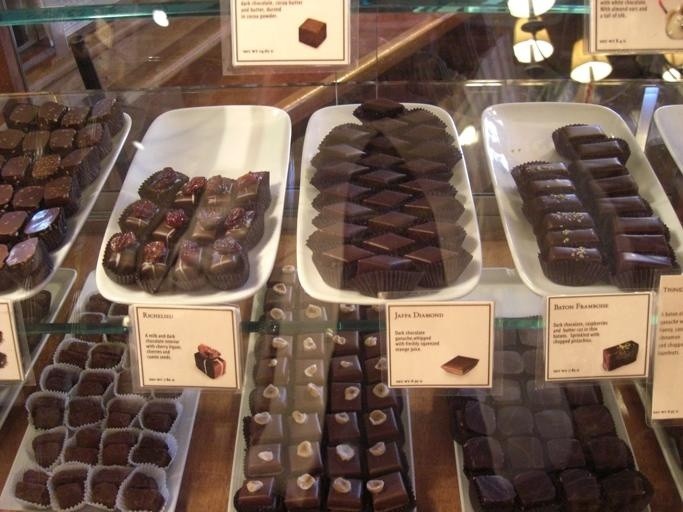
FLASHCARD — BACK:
[0,0,683,510]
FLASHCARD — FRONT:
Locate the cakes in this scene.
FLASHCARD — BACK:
[298,19,328,47]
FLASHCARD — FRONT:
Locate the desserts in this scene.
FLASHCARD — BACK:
[102,166,271,295]
[2,97,125,295]
[13,291,185,512]
[440,356,479,376]
[603,340,639,371]
[194,344,226,379]
[511,124,679,292]
[234,265,417,512]
[451,329,656,512]
[306,98,474,299]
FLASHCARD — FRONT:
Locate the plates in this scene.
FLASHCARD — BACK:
[651,101,683,178]
[628,378,682,512]
[228,288,417,512]
[452,376,641,511]
[0,267,210,512]
[295,100,484,306]
[97,104,294,307]
[0,111,130,307]
[479,100,683,295]
[0,268,76,430]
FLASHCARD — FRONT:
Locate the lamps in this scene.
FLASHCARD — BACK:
[506,0,559,33]
[510,16,555,77]
[568,38,613,89]
[660,60,682,85]
